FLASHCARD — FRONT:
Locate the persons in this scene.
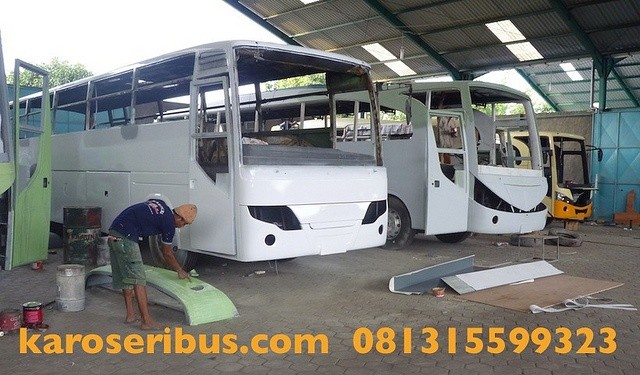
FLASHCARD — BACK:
[107,199,198,330]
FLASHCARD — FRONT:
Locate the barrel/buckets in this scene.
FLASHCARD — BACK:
[22,301,43,324]
[54,265,85,312]
[96,235,110,265]
[0,309,21,331]
[63,206,102,264]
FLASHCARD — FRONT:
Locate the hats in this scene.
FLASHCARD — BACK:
[174,204,198,224]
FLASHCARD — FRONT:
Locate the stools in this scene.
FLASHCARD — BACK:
[518,232,560,264]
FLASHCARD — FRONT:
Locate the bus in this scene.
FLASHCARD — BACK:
[3,39,52,277]
[14,38,398,266]
[154,80,552,252]
[496,131,606,226]
